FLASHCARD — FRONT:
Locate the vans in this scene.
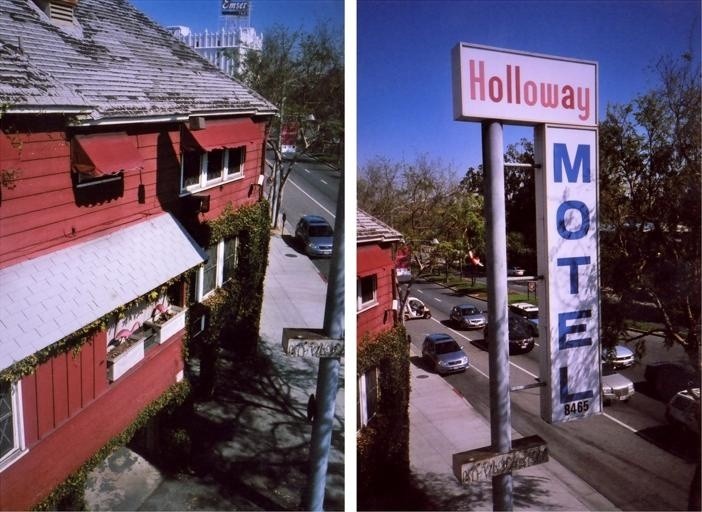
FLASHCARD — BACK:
[665,386,701,439]
[509,299,539,326]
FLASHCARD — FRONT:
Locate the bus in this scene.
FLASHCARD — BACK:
[395,243,414,283]
[268,118,298,161]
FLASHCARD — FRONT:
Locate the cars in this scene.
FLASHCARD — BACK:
[449,303,487,329]
[296,216,334,259]
[483,317,535,354]
[602,341,636,371]
[507,264,526,278]
[599,358,635,406]
[421,332,470,376]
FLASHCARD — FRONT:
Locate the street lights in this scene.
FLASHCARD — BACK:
[270,115,315,222]
[397,236,440,344]
